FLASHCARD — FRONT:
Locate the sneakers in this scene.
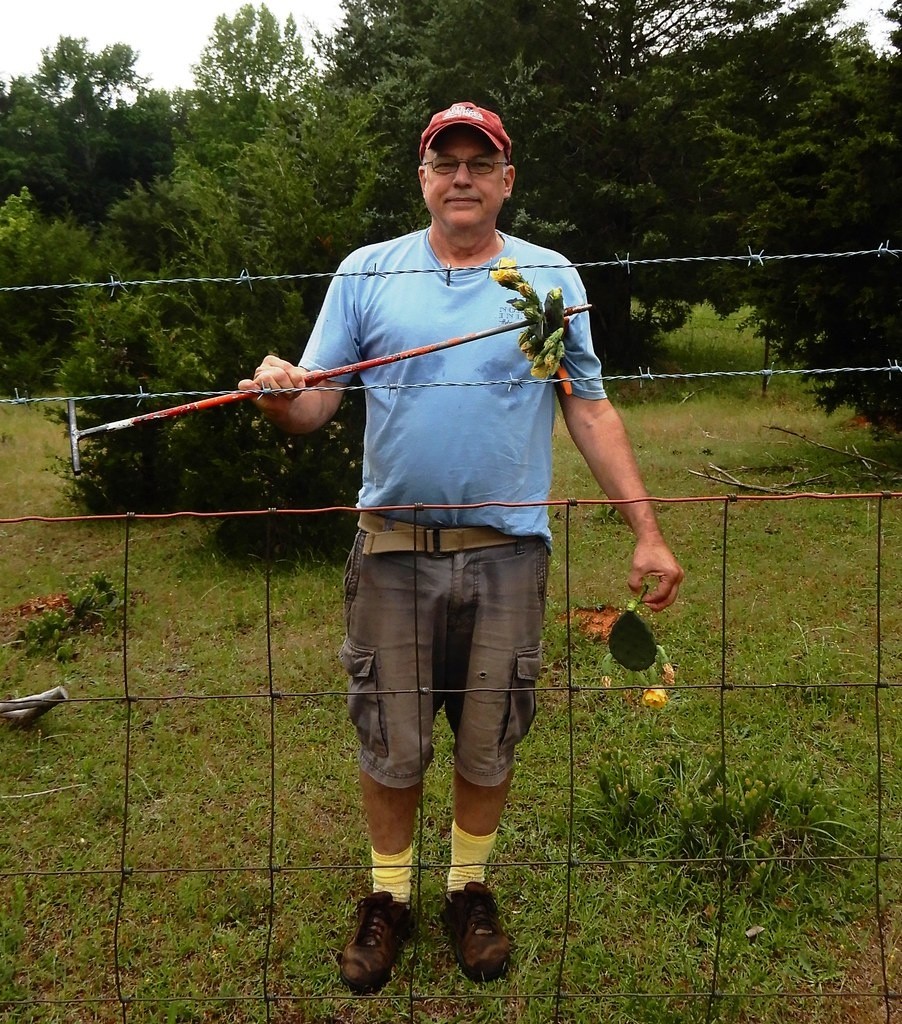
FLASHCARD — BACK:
[340,891,414,992]
[439,880,510,983]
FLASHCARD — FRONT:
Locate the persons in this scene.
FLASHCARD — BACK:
[236,98,684,996]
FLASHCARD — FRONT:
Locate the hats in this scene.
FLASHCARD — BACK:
[419,101,512,164]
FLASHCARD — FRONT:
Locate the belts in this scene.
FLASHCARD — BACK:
[356,511,537,556]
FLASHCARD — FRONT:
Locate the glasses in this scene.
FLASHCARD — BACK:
[422,155,508,174]
